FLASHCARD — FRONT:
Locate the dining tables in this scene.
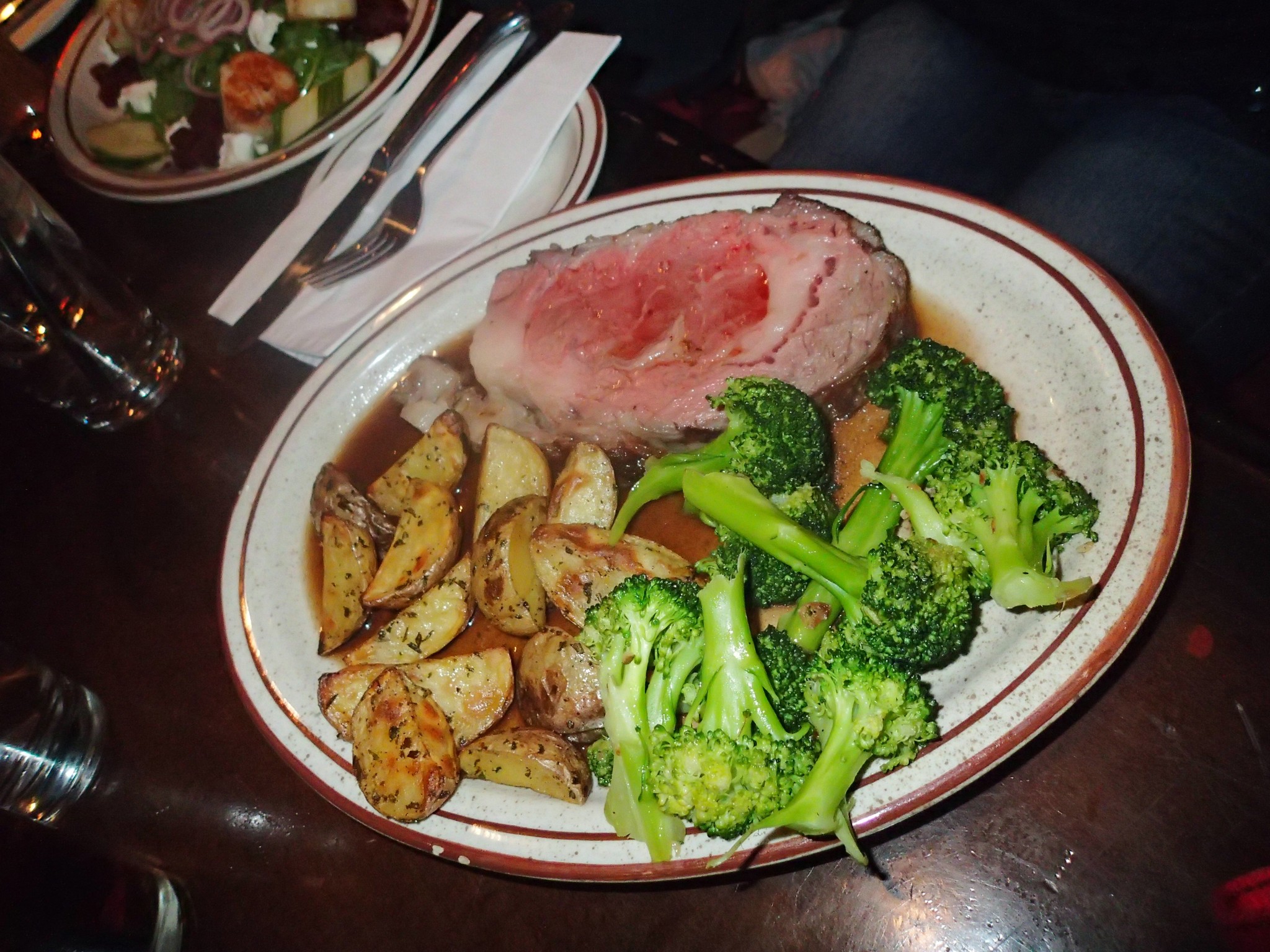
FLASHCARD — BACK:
[0,0,1269,952]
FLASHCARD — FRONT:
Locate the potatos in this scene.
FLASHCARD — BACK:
[307,407,695,825]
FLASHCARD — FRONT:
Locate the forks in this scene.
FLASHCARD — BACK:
[296,8,573,297]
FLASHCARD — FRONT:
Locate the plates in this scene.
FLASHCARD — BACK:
[218,166,1193,885]
[41,1,439,203]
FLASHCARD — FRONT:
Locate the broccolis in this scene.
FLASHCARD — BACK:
[577,334,1100,877]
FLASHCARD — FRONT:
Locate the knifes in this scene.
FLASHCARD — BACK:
[217,11,519,358]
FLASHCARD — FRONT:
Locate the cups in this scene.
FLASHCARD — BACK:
[0,634,107,822]
[0,804,183,951]
[0,152,180,436]
